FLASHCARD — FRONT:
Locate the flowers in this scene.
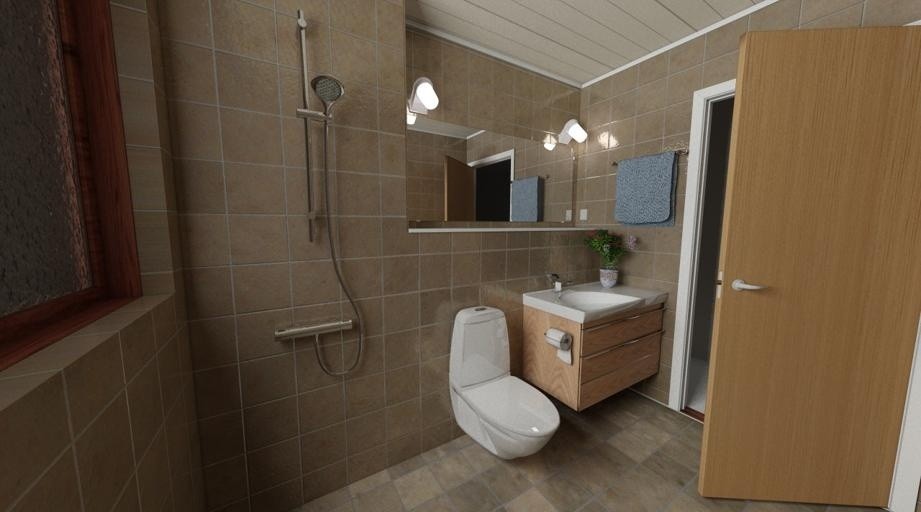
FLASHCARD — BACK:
[582,228,637,270]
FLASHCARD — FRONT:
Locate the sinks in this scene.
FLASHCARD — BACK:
[560,291,641,312]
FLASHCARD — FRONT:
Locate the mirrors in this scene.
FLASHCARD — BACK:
[405,108,578,228]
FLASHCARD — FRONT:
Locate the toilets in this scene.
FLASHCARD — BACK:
[448,305,560,461]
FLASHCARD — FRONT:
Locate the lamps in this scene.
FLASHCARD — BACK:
[559,118,588,145]
[407,76,440,115]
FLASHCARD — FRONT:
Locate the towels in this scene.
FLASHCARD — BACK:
[614,151,679,227]
[512,176,539,222]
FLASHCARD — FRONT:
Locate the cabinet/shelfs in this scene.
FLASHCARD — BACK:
[522,305,666,412]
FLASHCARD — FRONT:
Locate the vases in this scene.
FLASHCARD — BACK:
[599,269,619,289]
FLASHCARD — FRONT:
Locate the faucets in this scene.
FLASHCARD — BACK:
[554,279,576,293]
